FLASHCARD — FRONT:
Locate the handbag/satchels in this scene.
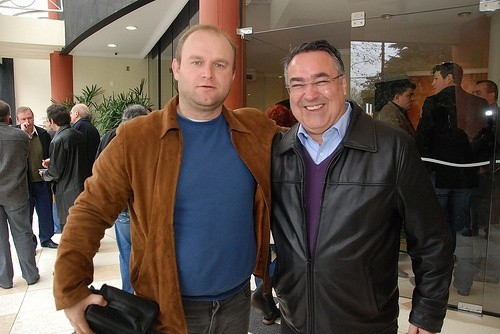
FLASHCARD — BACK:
[83,285,160,333]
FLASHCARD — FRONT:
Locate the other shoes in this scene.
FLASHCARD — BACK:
[462,229,477,236]
[251,287,282,318]
[398,269,410,278]
[262,308,283,325]
[28,274,41,285]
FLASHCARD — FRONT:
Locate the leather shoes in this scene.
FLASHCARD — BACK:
[41,239,59,248]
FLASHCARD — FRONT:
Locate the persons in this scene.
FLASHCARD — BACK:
[51,25,293,334]
[0,100,40,289]
[37,103,100,234]
[270,40,457,334]
[377,59,500,297]
[95,103,153,295]
[250,98,298,325]
[10,107,60,249]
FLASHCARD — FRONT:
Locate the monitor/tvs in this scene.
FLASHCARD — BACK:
[375,79,409,112]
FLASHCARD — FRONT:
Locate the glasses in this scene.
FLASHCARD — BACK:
[285,72,344,94]
